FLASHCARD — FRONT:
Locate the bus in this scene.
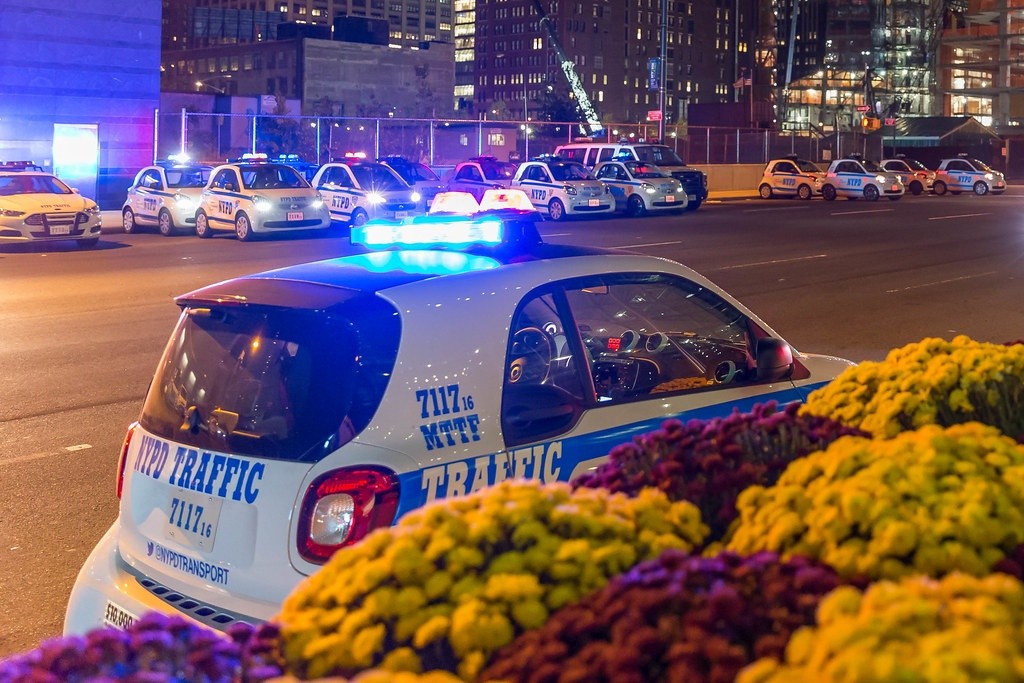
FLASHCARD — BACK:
[551,136,708,212]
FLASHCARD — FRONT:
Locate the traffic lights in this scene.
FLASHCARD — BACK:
[863,116,881,131]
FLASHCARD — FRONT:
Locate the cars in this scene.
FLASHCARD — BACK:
[267,154,322,187]
[878,155,938,195]
[0,160,101,249]
[64,177,861,655]
[758,154,825,201]
[375,154,449,210]
[821,154,905,202]
[195,153,332,241]
[588,151,690,218]
[513,153,616,223]
[441,153,517,204]
[929,153,1007,196]
[310,152,422,224]
[120,158,217,237]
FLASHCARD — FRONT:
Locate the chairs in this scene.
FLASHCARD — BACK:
[239,363,598,441]
